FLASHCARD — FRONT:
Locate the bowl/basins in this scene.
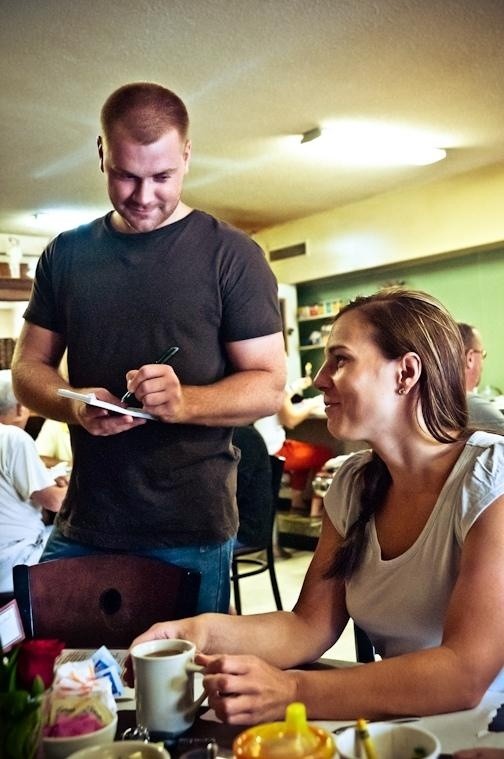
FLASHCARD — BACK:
[40,710,119,759]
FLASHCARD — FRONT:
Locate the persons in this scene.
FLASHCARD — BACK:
[0,384,63,605]
[11,82,288,623]
[455,320,504,435]
[120,286,503,727]
[225,424,277,549]
[32,417,74,470]
[257,371,328,521]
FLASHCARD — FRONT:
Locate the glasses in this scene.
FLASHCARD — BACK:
[474,349,487,360]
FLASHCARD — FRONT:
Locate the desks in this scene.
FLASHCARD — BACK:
[282,416,372,456]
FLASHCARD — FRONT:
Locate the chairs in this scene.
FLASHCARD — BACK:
[12,553,201,649]
[354,622,374,662]
[229,455,286,616]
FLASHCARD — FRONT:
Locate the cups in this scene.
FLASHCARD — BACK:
[335,722,442,759]
[65,740,171,759]
[129,638,212,735]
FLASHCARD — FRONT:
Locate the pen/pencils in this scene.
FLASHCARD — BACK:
[121,346,180,403]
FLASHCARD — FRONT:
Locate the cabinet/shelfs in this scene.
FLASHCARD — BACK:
[299,314,334,350]
